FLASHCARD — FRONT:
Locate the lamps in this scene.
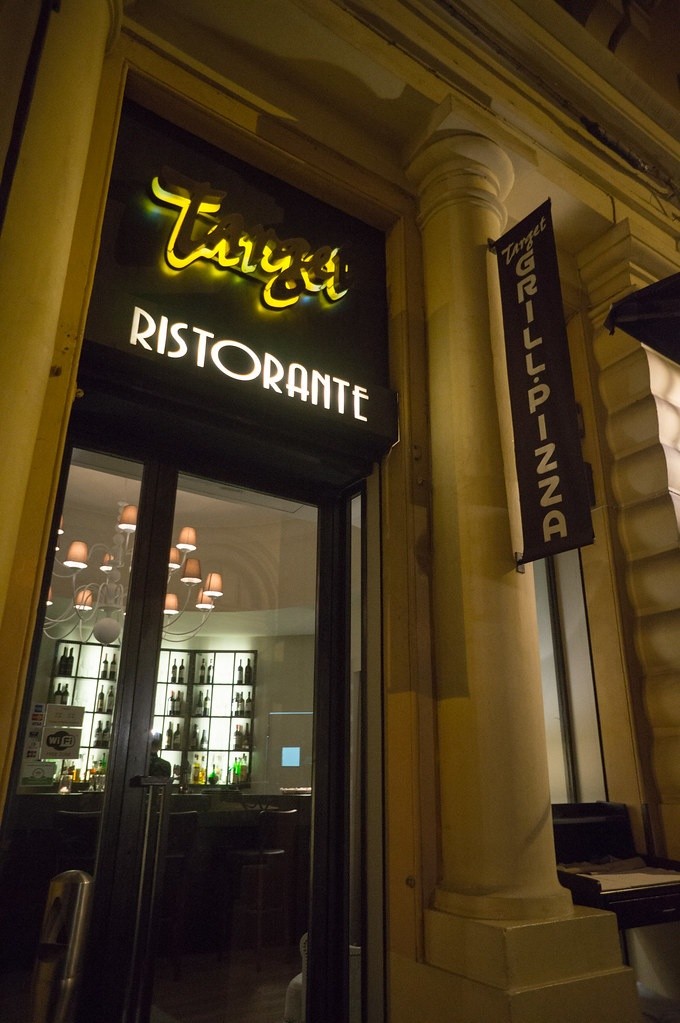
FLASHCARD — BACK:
[43,476,224,649]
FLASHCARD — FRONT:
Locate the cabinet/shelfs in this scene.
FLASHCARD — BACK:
[40,639,259,789]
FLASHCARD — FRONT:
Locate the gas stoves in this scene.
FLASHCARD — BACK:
[235,691,252,718]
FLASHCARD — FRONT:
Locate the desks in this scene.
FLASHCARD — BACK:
[552,855,680,967]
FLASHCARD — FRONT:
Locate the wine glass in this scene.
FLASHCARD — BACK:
[59,647,74,676]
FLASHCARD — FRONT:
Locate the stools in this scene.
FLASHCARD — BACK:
[149,811,199,858]
[54,809,102,867]
[223,808,305,972]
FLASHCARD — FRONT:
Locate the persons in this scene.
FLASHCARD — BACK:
[148,738,171,778]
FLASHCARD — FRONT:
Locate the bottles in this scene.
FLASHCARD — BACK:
[101,653,109,679]
[245,658,252,684]
[206,659,214,684]
[167,721,181,748]
[191,754,206,784]
[171,658,178,682]
[208,763,223,784]
[62,720,111,789]
[108,653,117,680]
[199,659,205,683]
[96,684,115,714]
[177,659,184,683]
[191,724,207,750]
[167,690,182,715]
[54,683,69,706]
[234,723,249,750]
[229,753,248,784]
[193,690,211,715]
[237,658,243,684]
